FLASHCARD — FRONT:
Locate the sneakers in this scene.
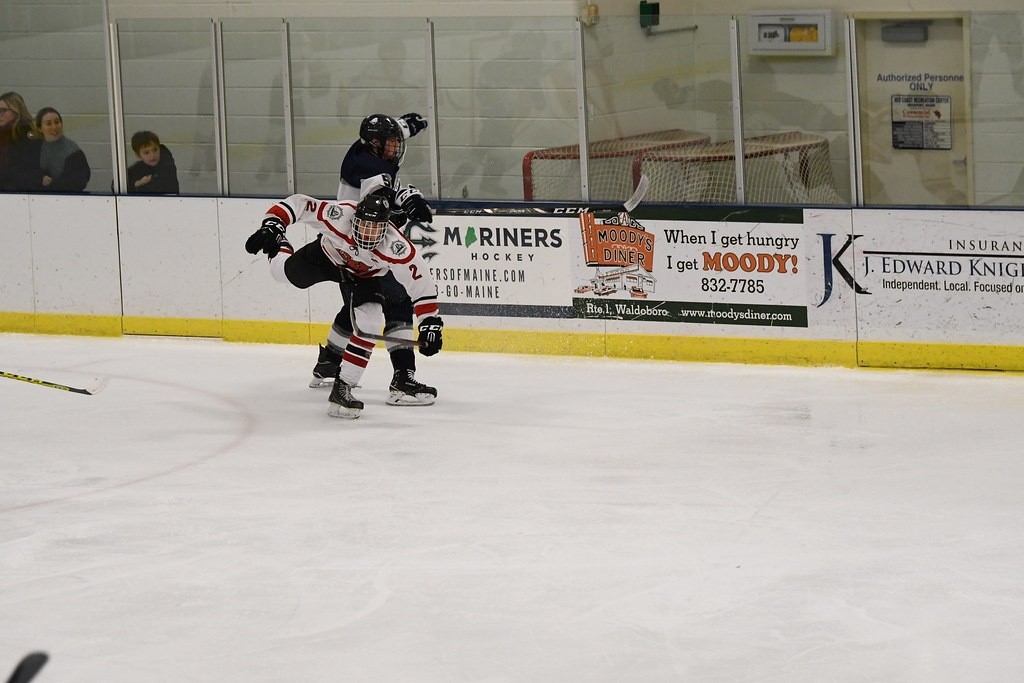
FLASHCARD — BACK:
[327,366,364,419]
[308,343,343,387]
[386,369,437,405]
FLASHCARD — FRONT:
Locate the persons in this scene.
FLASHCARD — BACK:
[25,106,90,193]
[283,112,438,397]
[246,194,443,411]
[0,91,38,193]
[111,130,181,195]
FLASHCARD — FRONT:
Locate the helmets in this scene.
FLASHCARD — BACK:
[359,114,401,156]
[354,194,390,223]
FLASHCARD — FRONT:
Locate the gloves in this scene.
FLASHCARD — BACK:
[417,316,444,357]
[401,195,432,223]
[400,112,428,137]
[245,217,286,259]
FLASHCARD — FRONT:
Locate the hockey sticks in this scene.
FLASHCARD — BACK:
[432,173,652,214]
[0,372,112,396]
[349,291,428,347]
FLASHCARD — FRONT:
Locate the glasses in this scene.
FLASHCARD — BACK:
[0,108,11,115]
